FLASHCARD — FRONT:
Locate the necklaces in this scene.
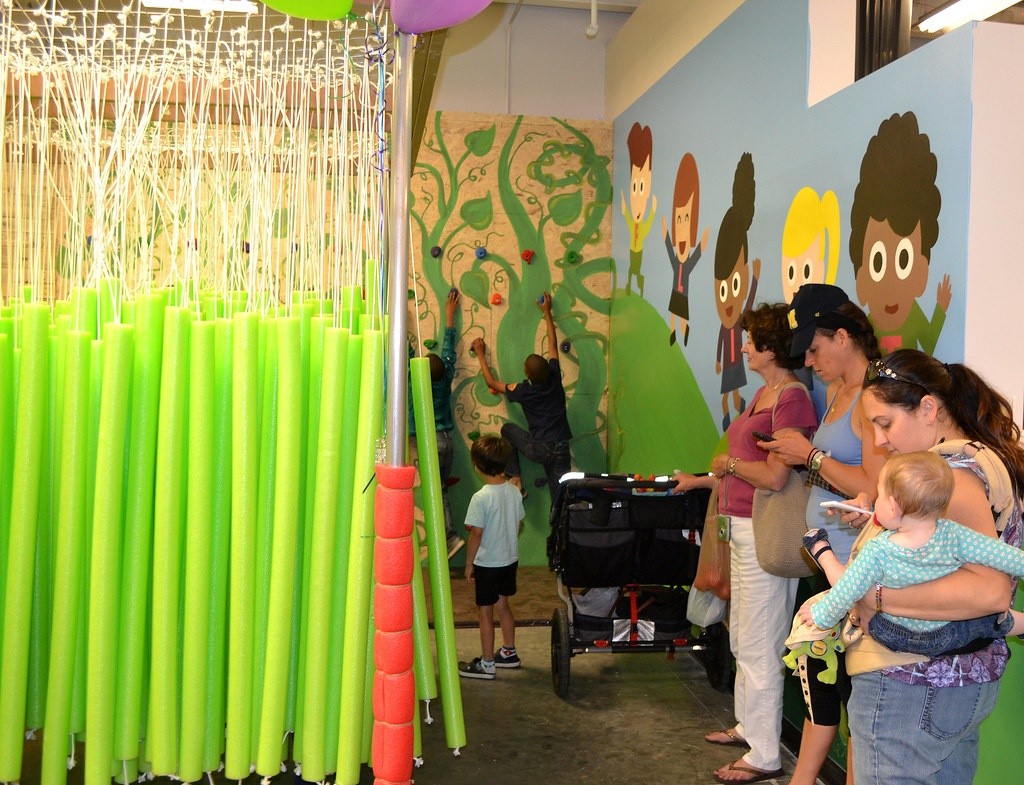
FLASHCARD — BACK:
[831,371,864,412]
[774,377,784,389]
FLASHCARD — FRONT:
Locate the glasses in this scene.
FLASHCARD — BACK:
[865,359,930,395]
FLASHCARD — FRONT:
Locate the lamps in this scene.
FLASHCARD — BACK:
[910,0,1023,35]
[585,0,599,35]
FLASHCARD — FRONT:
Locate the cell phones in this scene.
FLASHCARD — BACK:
[820,500,873,516]
[751,431,776,442]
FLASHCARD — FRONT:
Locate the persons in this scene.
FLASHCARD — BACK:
[471,292,577,501]
[798,450,1024,656]
[408,292,465,560]
[826,349,1024,785]
[672,302,817,785]
[457,435,526,680]
[757,283,888,785]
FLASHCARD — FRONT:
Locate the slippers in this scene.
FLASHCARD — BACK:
[714,760,785,785]
[705,730,752,749]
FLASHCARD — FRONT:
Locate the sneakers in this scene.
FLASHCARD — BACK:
[458,655,496,678]
[472,648,521,667]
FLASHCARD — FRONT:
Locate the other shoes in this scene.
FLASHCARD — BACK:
[519,487,527,497]
[447,536,465,559]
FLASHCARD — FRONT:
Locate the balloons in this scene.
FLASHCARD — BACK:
[390,0,492,34]
[259,0,353,21]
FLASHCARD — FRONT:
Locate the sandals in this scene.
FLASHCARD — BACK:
[803,528,839,572]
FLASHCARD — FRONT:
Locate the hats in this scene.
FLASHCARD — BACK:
[787,284,848,357]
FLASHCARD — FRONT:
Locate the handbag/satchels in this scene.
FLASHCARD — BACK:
[571,580,689,633]
[692,477,731,600]
[753,381,816,578]
[685,584,726,627]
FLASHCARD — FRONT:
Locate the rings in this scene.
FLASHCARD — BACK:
[850,615,859,622]
[849,522,851,527]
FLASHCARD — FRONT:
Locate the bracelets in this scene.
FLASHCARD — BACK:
[730,458,740,476]
[807,447,818,466]
[876,585,883,614]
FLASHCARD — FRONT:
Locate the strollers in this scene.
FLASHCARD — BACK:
[543,471,733,698]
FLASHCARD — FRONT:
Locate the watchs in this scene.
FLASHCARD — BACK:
[812,452,827,470]
